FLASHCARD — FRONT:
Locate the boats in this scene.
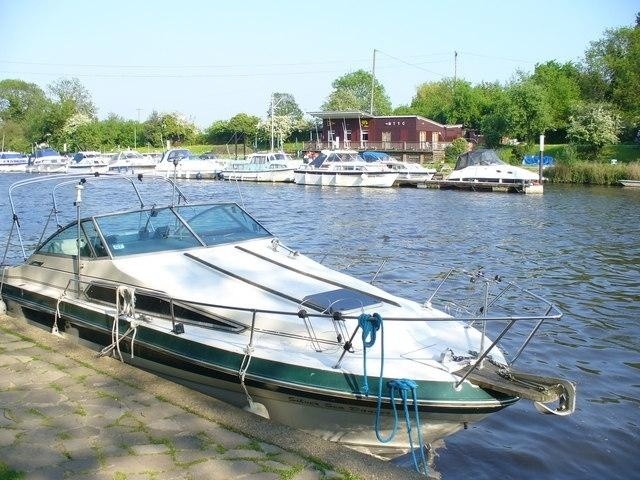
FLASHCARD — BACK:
[0,95,436,187]
[0,170,575,462]
[441,148,547,191]
